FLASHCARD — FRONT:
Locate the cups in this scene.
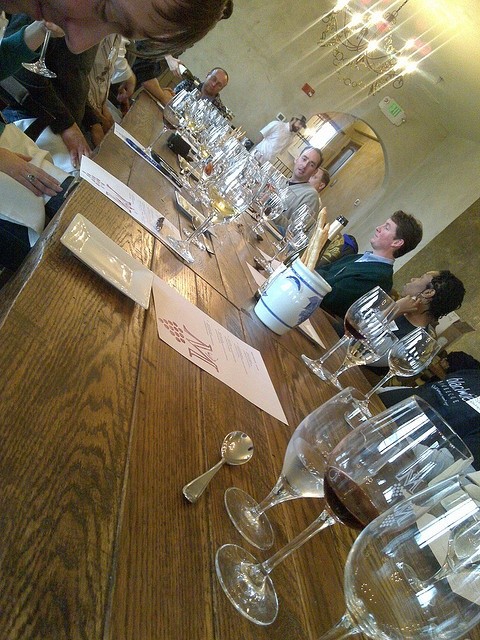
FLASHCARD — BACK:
[253,257,334,336]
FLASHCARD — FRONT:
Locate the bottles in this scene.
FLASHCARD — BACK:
[178,64,204,92]
[253,215,351,301]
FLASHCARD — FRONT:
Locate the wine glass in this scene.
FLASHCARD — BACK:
[168,164,286,261]
[346,328,444,411]
[300,286,401,383]
[181,85,290,164]
[312,469,480,639]
[140,88,198,160]
[225,392,390,549]
[328,322,396,390]
[413,503,480,599]
[216,386,472,624]
[254,204,315,274]
[22,27,61,82]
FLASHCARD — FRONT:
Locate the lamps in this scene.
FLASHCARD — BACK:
[316,0,422,99]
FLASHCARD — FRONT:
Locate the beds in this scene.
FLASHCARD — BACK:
[2,89,479,639]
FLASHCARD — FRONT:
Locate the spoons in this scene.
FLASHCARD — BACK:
[183,431,254,507]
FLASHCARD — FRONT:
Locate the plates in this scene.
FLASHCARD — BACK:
[60,211,155,311]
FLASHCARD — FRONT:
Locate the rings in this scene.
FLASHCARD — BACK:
[412,296,417,301]
[28,174,36,183]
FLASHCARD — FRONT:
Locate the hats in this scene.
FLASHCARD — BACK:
[292,113,306,128]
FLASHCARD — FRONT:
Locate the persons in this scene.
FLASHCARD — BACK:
[0,0,227,61]
[247,113,307,165]
[111,34,136,103]
[4,11,104,177]
[246,146,319,234]
[134,56,176,104]
[331,269,467,387]
[377,369,480,471]
[313,210,424,319]
[0,19,81,289]
[87,35,114,124]
[173,67,229,113]
[308,166,330,193]
